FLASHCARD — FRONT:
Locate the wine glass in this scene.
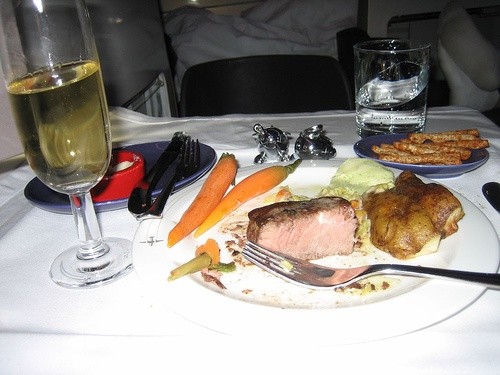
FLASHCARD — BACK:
[0,0,136,288]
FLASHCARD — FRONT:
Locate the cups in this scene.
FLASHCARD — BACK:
[72,150,145,206]
[353,40,432,140]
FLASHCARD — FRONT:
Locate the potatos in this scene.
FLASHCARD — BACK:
[366,169,465,260]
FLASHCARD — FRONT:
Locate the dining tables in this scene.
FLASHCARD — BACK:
[0,105,500,375]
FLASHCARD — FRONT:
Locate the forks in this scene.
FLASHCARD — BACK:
[239,241,500,289]
[136,139,200,222]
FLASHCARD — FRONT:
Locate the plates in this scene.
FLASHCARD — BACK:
[24,140,217,215]
[352,133,488,180]
[144,158,500,347]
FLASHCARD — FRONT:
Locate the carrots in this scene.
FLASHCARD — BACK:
[168,154,304,265]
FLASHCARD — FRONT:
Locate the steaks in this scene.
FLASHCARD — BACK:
[247,197,359,261]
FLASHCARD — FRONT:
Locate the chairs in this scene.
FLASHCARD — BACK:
[178,54,354,117]
